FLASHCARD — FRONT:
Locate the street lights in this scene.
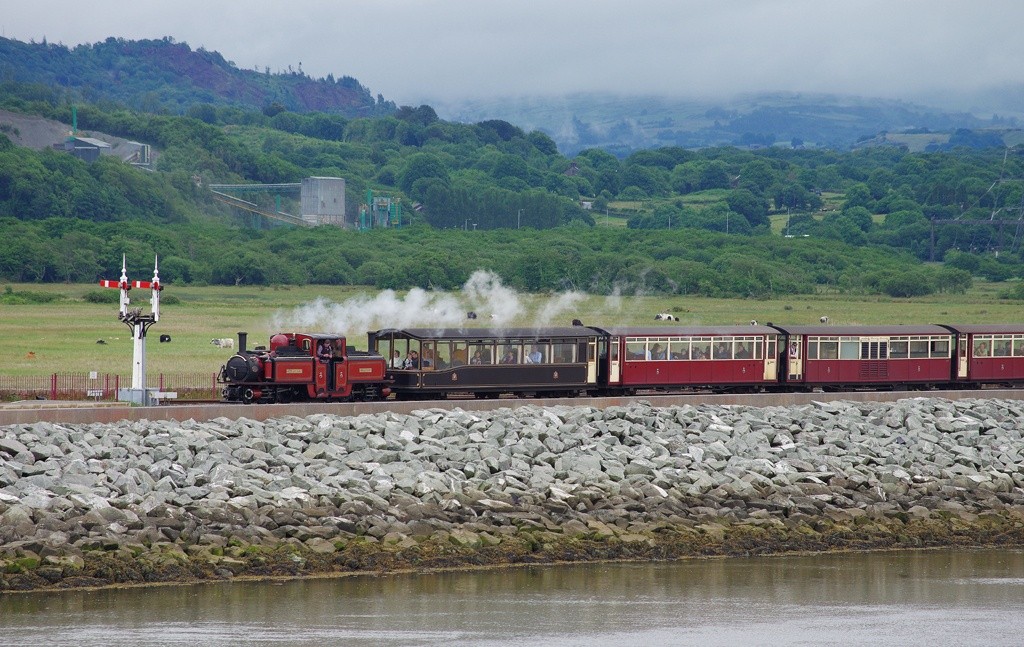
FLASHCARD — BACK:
[517,208,525,230]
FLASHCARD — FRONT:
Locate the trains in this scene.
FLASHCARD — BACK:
[216,322,1024,406]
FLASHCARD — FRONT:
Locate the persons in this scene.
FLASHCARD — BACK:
[790,341,798,359]
[975,342,1024,356]
[470,351,482,365]
[389,350,418,370]
[640,343,750,359]
[317,340,333,391]
[523,345,542,364]
[501,346,516,365]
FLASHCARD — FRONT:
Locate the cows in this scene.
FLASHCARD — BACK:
[572,319,584,327]
[160,335,171,343]
[467,312,477,318]
[820,316,827,323]
[654,314,679,321]
[750,320,758,326]
[210,338,233,349]
